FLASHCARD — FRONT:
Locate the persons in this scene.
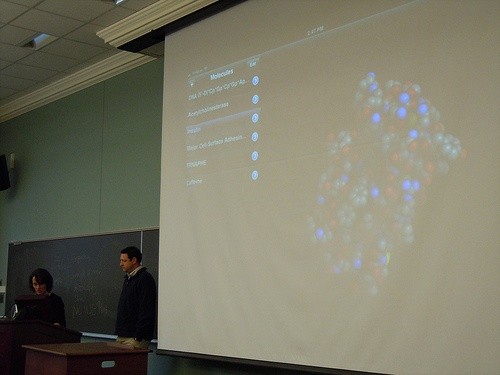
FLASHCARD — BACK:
[26,267,67,329]
[114,246,158,347]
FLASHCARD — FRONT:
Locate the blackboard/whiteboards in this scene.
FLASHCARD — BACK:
[5,225,160,344]
